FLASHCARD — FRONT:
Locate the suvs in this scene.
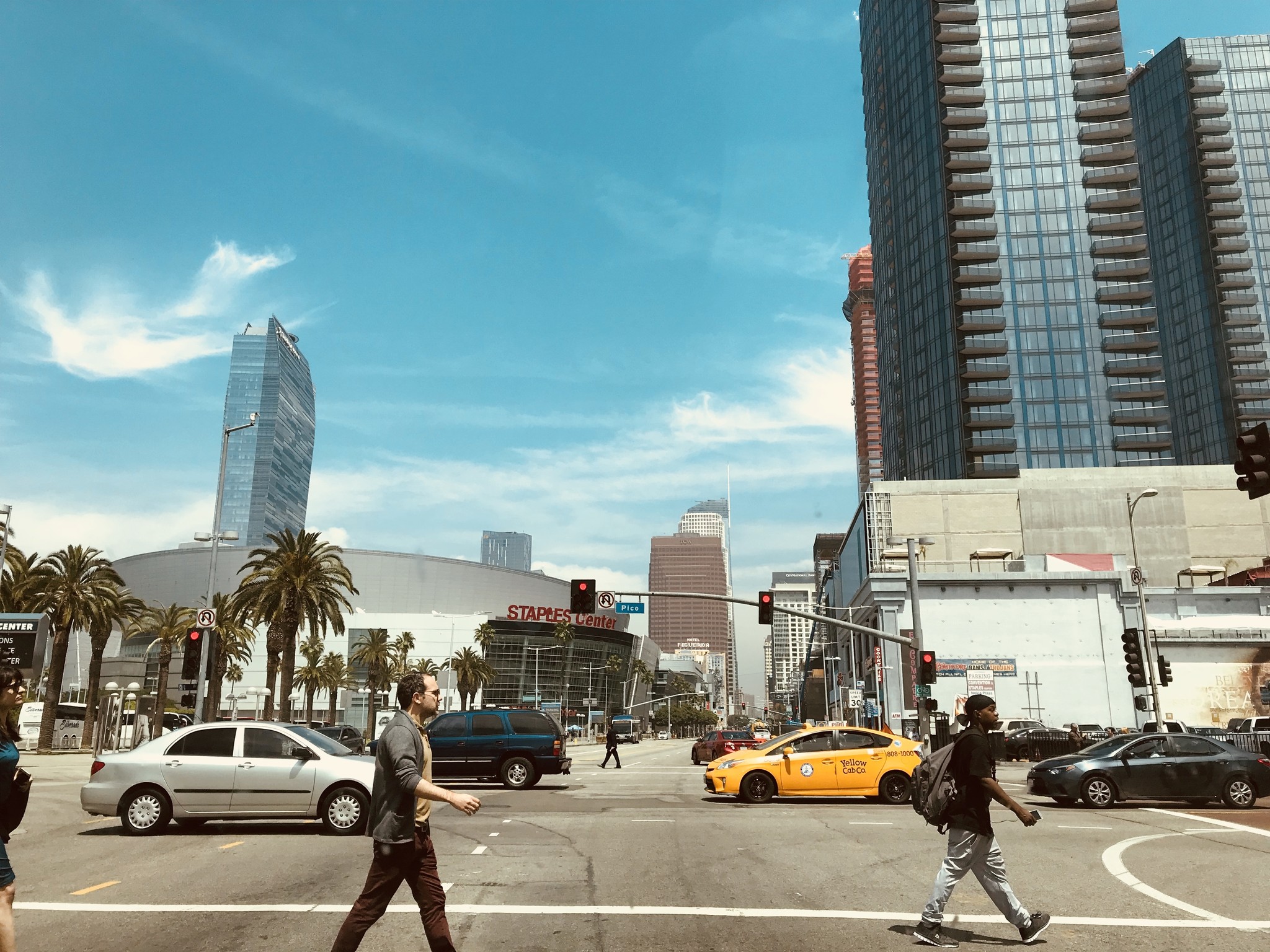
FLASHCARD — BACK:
[366,708,572,790]
[313,725,366,753]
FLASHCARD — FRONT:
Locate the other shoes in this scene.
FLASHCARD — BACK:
[614,767,621,769]
[597,764,605,769]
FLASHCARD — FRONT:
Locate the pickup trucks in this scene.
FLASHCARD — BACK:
[753,728,772,740]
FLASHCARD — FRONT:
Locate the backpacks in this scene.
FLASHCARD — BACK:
[912,728,996,836]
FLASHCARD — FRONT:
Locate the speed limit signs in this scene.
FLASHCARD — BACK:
[848,690,863,709]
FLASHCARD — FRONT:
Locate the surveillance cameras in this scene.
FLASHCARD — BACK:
[255,412,260,417]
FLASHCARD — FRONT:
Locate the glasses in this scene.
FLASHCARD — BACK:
[418,690,440,697]
[4,681,23,693]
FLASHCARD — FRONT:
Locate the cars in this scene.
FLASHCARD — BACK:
[1027,731,1270,808]
[657,731,668,740]
[991,714,1270,759]
[691,730,760,765]
[704,723,926,804]
[79,721,377,834]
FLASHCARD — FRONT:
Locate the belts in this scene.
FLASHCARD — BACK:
[416,826,429,832]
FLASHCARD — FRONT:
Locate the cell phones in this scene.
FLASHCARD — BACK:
[1029,809,1044,821]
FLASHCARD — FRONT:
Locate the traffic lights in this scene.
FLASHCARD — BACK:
[789,717,793,721]
[757,592,774,625]
[918,651,937,685]
[742,703,746,710]
[1135,695,1147,711]
[1157,655,1173,687]
[181,694,195,707]
[795,706,799,713]
[182,628,204,681]
[764,707,768,714]
[570,579,595,613]
[1120,628,1146,688]
[1233,421,1270,498]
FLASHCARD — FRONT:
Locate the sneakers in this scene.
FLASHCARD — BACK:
[913,920,960,947]
[1019,911,1050,942]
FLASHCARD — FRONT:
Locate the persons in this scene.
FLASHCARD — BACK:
[330,673,482,952]
[912,694,1051,949]
[1068,723,1087,753]
[570,727,576,742]
[908,727,920,742]
[801,735,831,751]
[1119,727,1130,734]
[1143,741,1161,759]
[568,730,581,737]
[1104,727,1115,740]
[0,665,28,951]
[596,723,622,768]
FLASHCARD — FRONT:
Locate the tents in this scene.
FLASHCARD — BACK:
[566,724,585,737]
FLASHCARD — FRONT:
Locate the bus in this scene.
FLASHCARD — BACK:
[18,703,99,749]
[611,715,641,744]
[105,709,331,750]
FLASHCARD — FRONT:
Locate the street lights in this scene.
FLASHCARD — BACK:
[1126,487,1168,732]
[191,411,259,723]
[770,605,892,732]
[522,644,564,711]
[885,536,935,757]
[42,678,390,753]
[576,663,716,742]
[0,503,13,579]
[431,610,493,713]
